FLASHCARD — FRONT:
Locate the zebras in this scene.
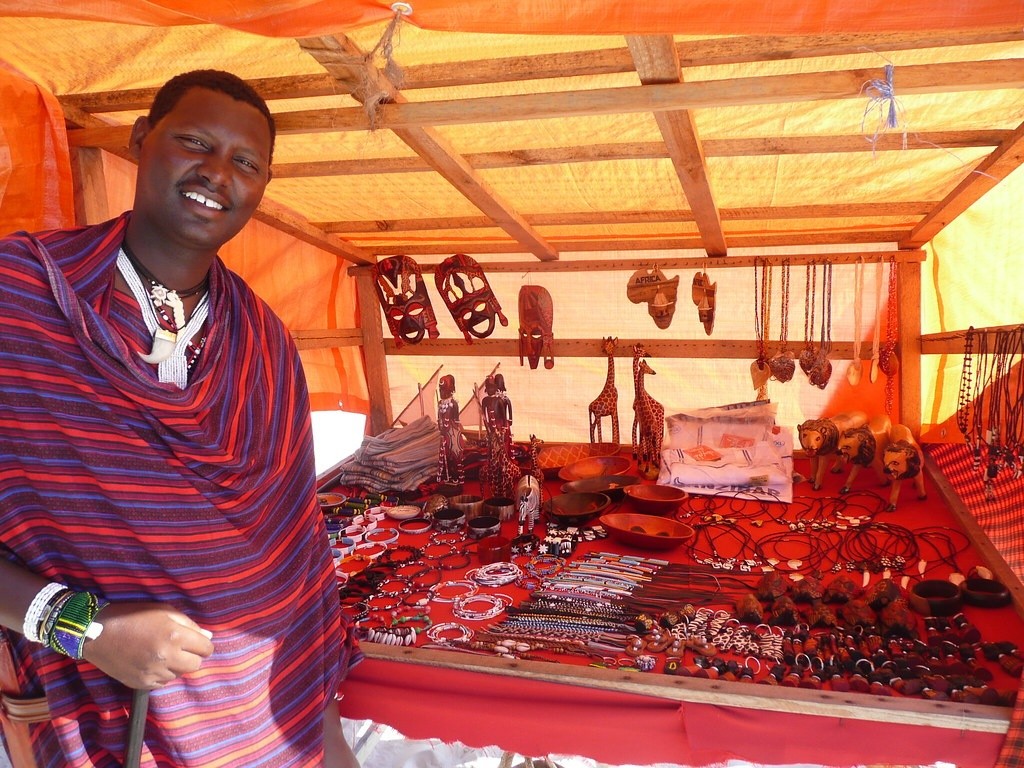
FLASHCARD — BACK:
[515,475,540,536]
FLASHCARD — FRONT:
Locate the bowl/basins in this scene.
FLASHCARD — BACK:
[542,492,610,526]
[560,475,641,499]
[541,442,621,477]
[559,456,632,481]
[623,484,689,515]
[599,513,694,549]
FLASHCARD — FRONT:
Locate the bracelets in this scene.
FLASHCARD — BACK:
[23,582,110,660]
[324,494,566,645]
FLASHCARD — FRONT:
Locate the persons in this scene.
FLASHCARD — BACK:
[436,375,464,485]
[1,69,365,768]
[481,374,514,492]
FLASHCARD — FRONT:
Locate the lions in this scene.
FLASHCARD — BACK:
[797,410,927,513]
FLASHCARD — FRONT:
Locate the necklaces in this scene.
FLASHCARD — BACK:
[956,325,1024,499]
[675,489,992,586]
[848,255,898,414]
[124,241,210,391]
[420,550,764,663]
[750,256,832,402]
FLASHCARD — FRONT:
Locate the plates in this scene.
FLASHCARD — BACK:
[318,493,346,511]
[387,506,421,519]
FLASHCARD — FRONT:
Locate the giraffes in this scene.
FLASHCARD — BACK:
[632,343,665,474]
[529,433,544,511]
[588,336,619,446]
